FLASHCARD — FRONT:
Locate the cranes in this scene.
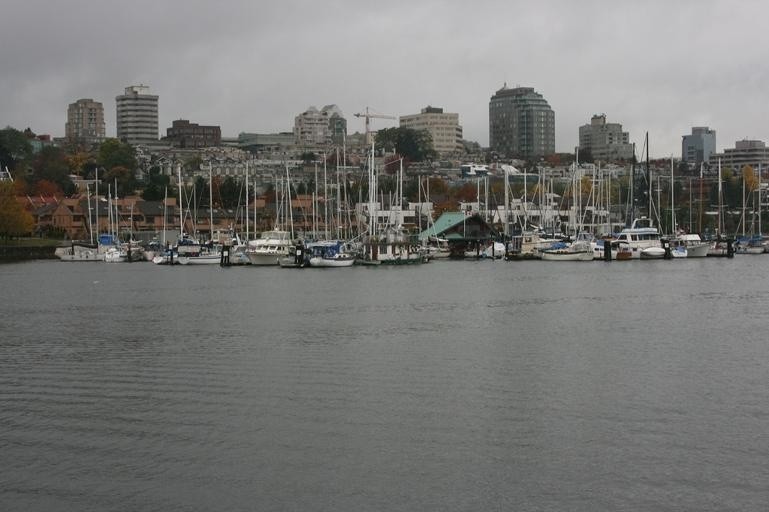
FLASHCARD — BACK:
[353,106,397,149]
